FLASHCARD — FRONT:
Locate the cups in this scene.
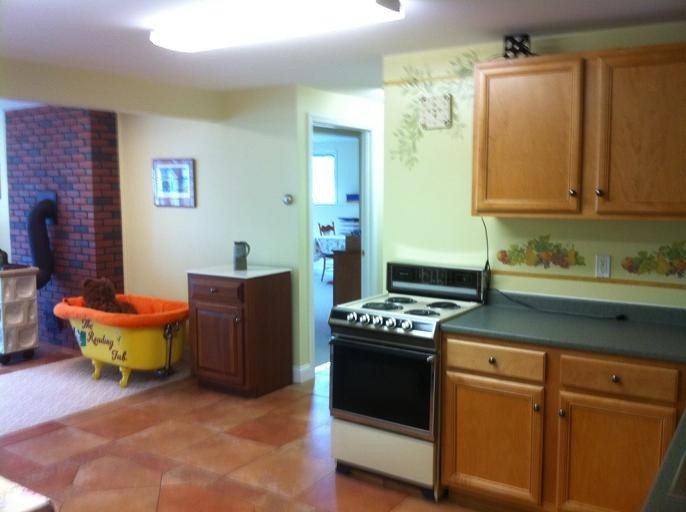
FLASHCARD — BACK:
[233,240,250,270]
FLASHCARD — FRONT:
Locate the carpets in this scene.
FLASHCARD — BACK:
[2,348,191,435]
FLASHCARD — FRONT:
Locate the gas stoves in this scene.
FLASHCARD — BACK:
[327,294,484,333]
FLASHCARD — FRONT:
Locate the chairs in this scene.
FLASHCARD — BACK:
[319,223,337,282]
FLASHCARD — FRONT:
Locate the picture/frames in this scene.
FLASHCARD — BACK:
[152,159,195,208]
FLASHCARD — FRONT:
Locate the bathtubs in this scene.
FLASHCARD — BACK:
[52,292,193,388]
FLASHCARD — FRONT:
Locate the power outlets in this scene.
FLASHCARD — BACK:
[596,255,611,279]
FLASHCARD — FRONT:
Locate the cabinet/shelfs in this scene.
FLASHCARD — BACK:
[441,294,685,511]
[472,43,686,220]
[189,265,292,398]
[332,248,360,307]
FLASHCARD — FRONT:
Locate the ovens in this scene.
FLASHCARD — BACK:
[327,327,437,489]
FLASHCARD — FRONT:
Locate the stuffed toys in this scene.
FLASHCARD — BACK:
[83,276,137,314]
